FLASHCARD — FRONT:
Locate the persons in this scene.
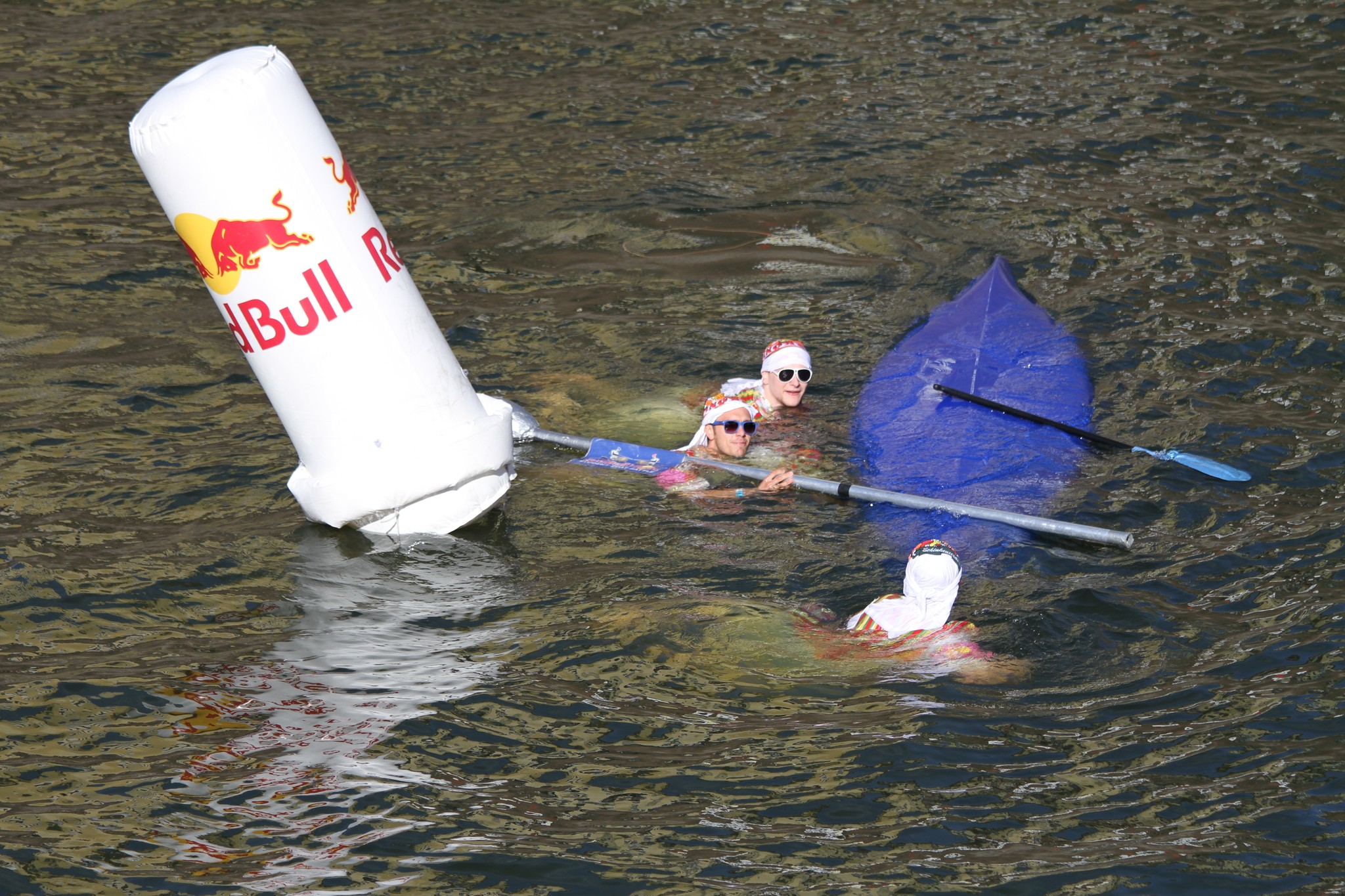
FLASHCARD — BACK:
[844,539,1029,686]
[657,396,794,503]
[719,339,813,422]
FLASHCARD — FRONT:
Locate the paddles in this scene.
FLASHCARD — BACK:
[933,382,1251,482]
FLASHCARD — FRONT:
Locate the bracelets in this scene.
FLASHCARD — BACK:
[734,486,744,499]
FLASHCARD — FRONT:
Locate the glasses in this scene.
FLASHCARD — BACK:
[772,368,813,383]
[710,421,757,435]
